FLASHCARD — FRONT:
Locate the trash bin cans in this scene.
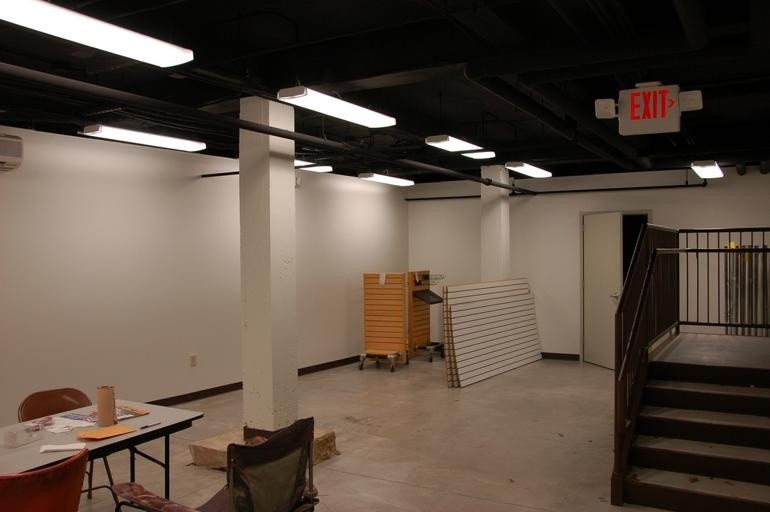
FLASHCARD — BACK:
[724,245,770,337]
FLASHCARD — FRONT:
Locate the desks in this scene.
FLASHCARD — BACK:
[0,397,205,503]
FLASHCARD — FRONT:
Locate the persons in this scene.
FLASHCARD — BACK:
[243,436,268,446]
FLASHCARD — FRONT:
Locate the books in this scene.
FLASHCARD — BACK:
[24,402,150,453]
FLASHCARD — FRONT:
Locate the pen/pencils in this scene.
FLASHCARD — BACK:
[140,422,162,429]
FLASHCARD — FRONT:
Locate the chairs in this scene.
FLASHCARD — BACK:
[0,386,120,512]
[109,417,318,512]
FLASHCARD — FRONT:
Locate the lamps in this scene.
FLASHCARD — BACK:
[357,135,416,187]
[425,92,497,160]
[276,25,398,130]
[84,121,207,154]
[505,124,553,180]
